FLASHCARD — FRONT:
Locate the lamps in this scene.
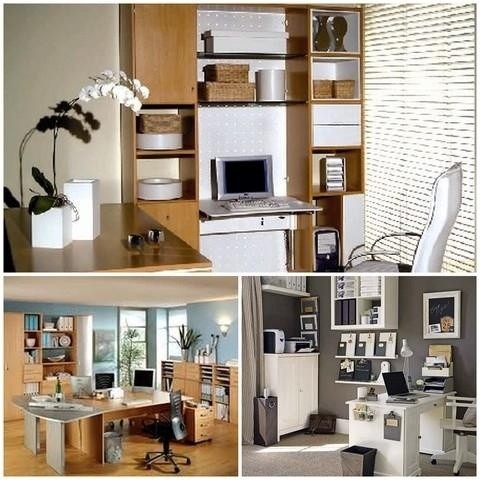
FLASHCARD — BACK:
[400,339,413,391]
[215,317,234,338]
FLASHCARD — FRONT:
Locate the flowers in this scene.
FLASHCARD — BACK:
[28,68,154,224]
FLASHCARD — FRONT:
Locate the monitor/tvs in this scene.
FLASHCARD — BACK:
[94,371,117,392]
[215,155,274,201]
[70,376,92,399]
[133,368,156,393]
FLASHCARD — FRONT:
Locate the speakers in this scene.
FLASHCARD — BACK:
[263,328,285,354]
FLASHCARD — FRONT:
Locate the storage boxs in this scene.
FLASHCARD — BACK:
[201,30,291,56]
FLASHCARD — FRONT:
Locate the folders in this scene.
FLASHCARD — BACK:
[25,316,38,330]
[349,300,356,324]
[335,300,343,325]
[57,316,73,331]
[343,300,349,325]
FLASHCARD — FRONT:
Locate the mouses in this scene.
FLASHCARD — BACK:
[292,200,303,206]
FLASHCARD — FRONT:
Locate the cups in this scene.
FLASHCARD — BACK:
[148,229,164,247]
[356,387,368,400]
[127,233,144,250]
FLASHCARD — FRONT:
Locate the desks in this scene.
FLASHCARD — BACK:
[345,389,457,476]
[10,386,194,475]
[4,202,212,272]
[200,196,323,272]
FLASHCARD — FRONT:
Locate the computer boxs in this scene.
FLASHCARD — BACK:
[312,227,341,272]
[254,396,277,447]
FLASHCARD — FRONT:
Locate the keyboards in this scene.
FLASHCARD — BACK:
[224,199,291,212]
[122,398,153,406]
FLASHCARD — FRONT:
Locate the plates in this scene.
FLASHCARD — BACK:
[59,336,72,347]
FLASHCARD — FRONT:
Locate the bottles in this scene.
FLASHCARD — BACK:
[264,387,269,400]
[55,380,62,402]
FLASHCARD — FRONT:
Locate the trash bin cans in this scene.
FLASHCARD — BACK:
[105,432,123,463]
[340,445,377,476]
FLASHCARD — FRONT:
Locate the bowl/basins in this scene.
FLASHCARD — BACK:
[25,338,36,346]
[44,322,55,329]
[44,376,59,381]
[47,357,62,362]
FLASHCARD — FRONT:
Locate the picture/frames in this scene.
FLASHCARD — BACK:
[423,291,461,339]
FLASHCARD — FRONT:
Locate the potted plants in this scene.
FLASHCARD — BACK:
[169,324,201,361]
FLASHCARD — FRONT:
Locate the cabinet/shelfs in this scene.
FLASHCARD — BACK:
[159,360,173,392]
[121,104,198,255]
[334,354,398,386]
[199,365,238,423]
[261,354,322,442]
[286,5,364,101]
[422,362,455,393]
[41,315,78,379]
[120,5,196,104]
[185,406,213,444]
[5,313,43,423]
[308,101,366,268]
[192,4,308,101]
[330,276,399,332]
[173,363,200,425]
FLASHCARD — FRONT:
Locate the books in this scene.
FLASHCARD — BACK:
[333,277,383,327]
[262,276,306,292]
[162,361,229,420]
[23,314,76,364]
[422,356,451,393]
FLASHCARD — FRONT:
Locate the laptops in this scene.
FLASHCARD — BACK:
[381,371,430,399]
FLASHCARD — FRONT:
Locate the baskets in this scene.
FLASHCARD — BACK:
[139,114,182,133]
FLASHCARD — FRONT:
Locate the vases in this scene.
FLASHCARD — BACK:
[63,178,101,239]
[27,204,72,249]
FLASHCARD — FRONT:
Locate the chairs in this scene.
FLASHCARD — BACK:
[120,367,159,428]
[344,162,463,273]
[429,397,476,475]
[93,371,117,430]
[144,389,191,474]
